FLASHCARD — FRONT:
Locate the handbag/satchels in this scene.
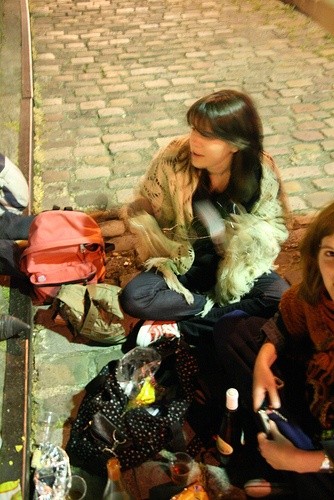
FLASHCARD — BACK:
[69,333,213,477]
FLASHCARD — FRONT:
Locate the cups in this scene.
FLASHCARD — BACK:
[169,452,193,485]
[35,411,59,444]
[66,475,88,500]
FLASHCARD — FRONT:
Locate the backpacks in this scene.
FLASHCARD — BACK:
[19,210,115,285]
[51,282,141,345]
[0,152,29,216]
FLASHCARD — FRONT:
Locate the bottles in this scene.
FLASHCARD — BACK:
[216,388,246,470]
[102,457,133,500]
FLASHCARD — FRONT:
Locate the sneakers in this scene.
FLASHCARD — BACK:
[136,320,181,347]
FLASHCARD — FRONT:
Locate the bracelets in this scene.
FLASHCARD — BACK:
[319,452,334,474]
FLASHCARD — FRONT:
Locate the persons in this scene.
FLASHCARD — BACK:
[117,89,293,346]
[211,202,334,500]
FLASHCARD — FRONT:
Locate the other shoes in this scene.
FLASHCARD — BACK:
[244,479,284,497]
